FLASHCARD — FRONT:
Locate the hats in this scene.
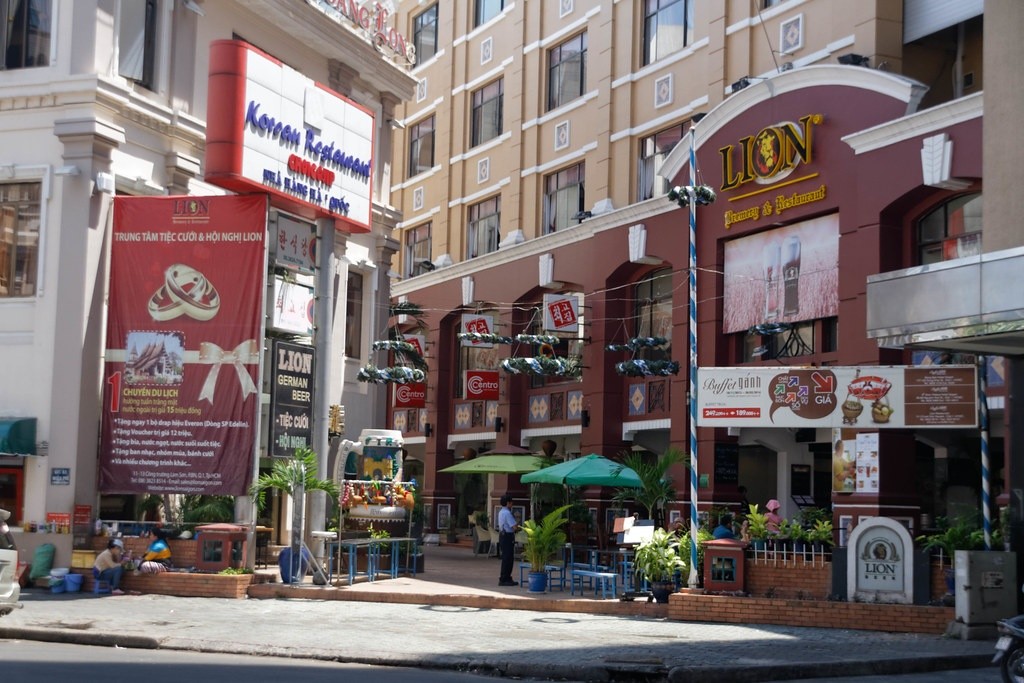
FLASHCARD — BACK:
[110,539,124,549]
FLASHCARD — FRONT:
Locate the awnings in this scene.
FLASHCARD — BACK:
[0,417,37,457]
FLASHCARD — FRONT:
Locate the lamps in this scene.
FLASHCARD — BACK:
[419,261,435,271]
[581,410,590,427]
[495,417,503,432]
[571,211,593,220]
[425,423,432,438]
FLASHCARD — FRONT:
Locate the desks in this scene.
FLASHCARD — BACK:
[588,548,635,589]
[558,544,599,588]
[326,537,417,584]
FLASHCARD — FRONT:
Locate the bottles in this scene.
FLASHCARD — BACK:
[129,550,134,563]
[24,519,70,534]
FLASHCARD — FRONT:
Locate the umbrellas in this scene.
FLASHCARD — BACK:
[436,443,559,491]
[521,452,666,544]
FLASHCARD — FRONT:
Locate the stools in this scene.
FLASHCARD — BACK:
[93,579,111,595]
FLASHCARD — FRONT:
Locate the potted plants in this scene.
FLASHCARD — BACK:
[633,528,685,602]
[249,448,340,581]
[440,530,455,543]
[923,525,985,595]
[746,505,834,559]
[517,504,574,591]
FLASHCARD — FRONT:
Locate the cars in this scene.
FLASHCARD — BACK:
[0,508,25,618]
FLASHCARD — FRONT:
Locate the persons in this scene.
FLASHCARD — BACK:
[712,514,733,538]
[134,527,172,575]
[726,485,749,513]
[92,539,125,594]
[498,495,519,586]
[833,439,856,491]
[764,509,781,530]
[740,520,750,541]
[0,509,16,550]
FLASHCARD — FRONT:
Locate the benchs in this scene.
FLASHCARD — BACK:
[519,558,636,600]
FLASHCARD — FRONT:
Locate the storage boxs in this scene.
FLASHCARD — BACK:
[71,549,100,569]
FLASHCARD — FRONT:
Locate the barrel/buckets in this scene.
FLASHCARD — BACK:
[50,567,86,593]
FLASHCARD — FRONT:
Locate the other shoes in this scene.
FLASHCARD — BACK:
[499,581,518,586]
[112,589,125,595]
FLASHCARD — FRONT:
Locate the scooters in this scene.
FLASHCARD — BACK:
[990,615,1024,683]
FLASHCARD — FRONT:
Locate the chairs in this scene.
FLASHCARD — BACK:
[486,524,499,559]
[473,525,490,558]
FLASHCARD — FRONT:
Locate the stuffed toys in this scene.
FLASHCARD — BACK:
[342,484,414,509]
